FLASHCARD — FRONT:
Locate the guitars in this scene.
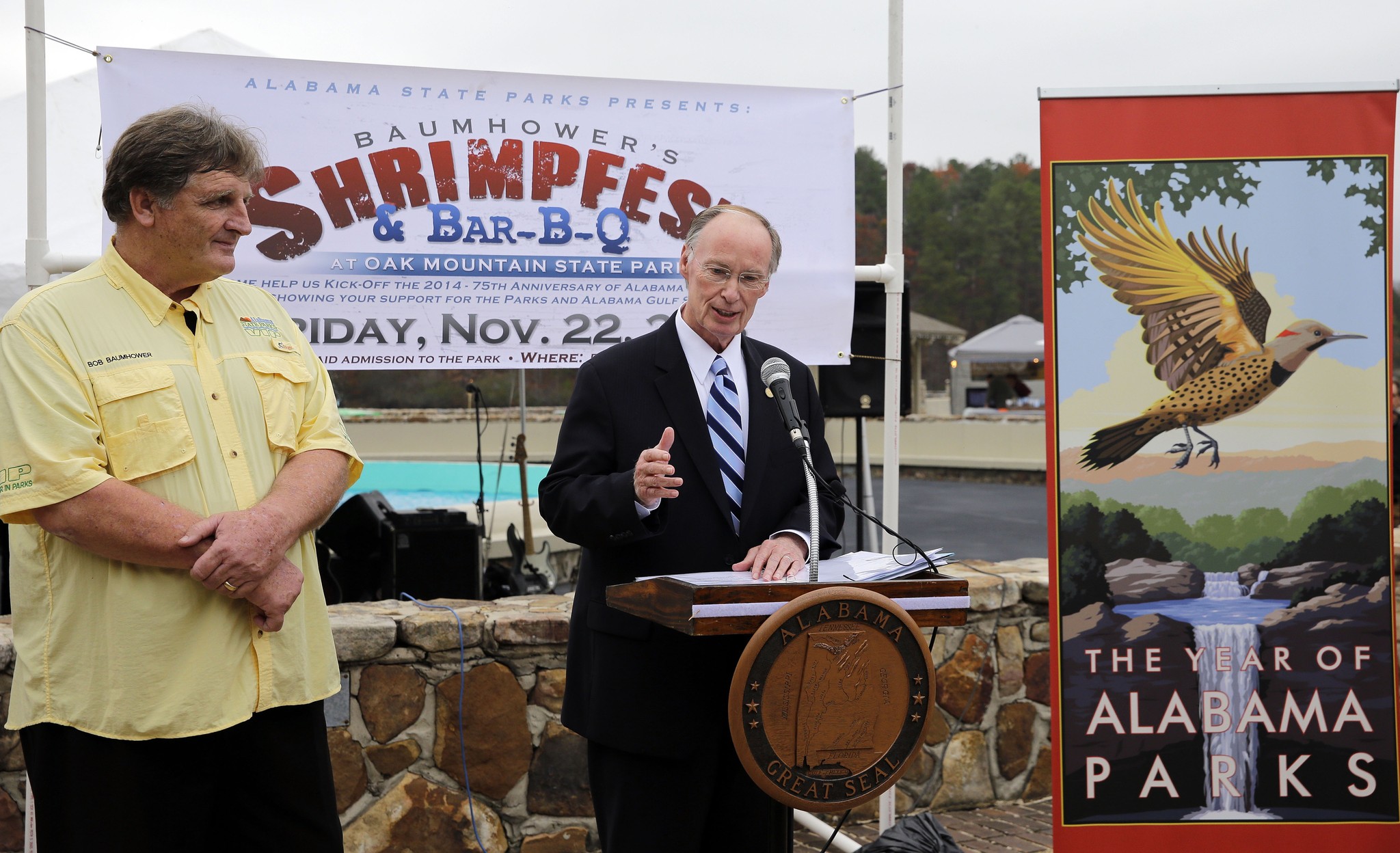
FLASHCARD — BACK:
[508,433,557,595]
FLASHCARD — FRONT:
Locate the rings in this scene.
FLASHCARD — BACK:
[223,581,238,591]
[783,556,792,562]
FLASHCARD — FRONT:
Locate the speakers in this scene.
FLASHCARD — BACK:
[309,487,399,607]
[818,279,913,418]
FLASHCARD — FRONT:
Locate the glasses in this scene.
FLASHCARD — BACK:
[691,246,767,290]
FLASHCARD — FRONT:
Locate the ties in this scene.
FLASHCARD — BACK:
[707,354,745,536]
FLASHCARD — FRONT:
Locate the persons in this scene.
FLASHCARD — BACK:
[983,373,1031,410]
[0,95,364,853]
[538,204,847,853]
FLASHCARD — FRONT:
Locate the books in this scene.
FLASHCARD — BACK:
[636,547,960,584]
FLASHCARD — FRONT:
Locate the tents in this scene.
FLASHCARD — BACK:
[909,309,968,414]
[947,313,1044,414]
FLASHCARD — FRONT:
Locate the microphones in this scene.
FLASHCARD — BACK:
[465,383,481,393]
[761,356,806,457]
